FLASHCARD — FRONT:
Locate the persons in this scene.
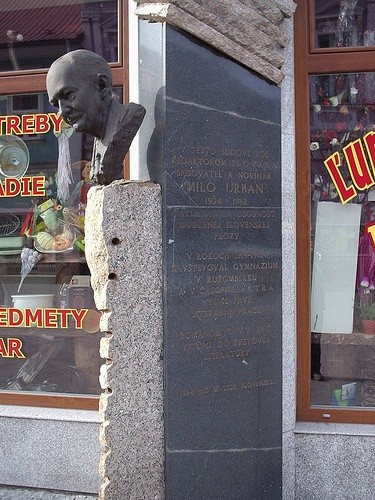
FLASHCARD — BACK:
[45,49,146,186]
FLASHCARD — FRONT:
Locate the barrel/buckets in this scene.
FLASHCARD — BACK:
[10,294,56,327]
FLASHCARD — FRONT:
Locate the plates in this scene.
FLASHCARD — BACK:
[33,227,76,254]
